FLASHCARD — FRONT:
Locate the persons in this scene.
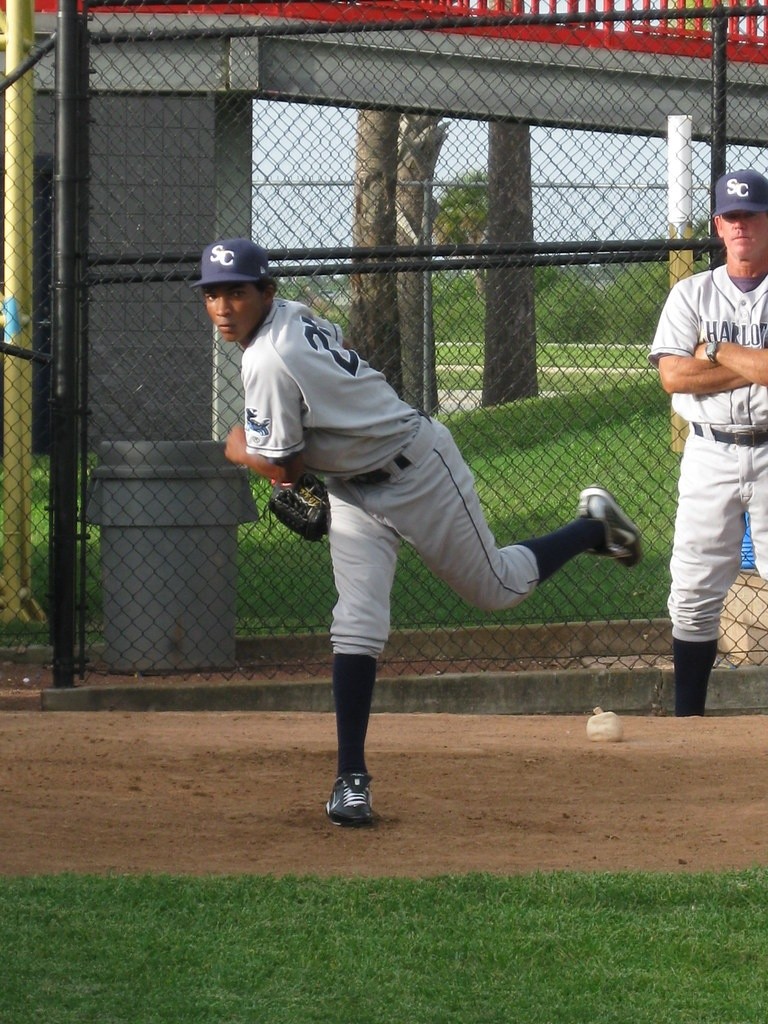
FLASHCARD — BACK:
[648,170,768,718]
[190,239,644,825]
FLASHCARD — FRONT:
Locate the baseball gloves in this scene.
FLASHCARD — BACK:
[260,473,332,544]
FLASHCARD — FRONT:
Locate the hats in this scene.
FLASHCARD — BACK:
[712,169,768,218]
[189,239,269,289]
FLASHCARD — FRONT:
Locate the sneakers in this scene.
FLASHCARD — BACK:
[325,772,372,827]
[578,485,640,567]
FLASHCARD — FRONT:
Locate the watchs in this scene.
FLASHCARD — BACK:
[705,340,719,362]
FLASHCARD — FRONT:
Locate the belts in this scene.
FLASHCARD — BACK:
[343,408,432,485]
[692,423,768,447]
